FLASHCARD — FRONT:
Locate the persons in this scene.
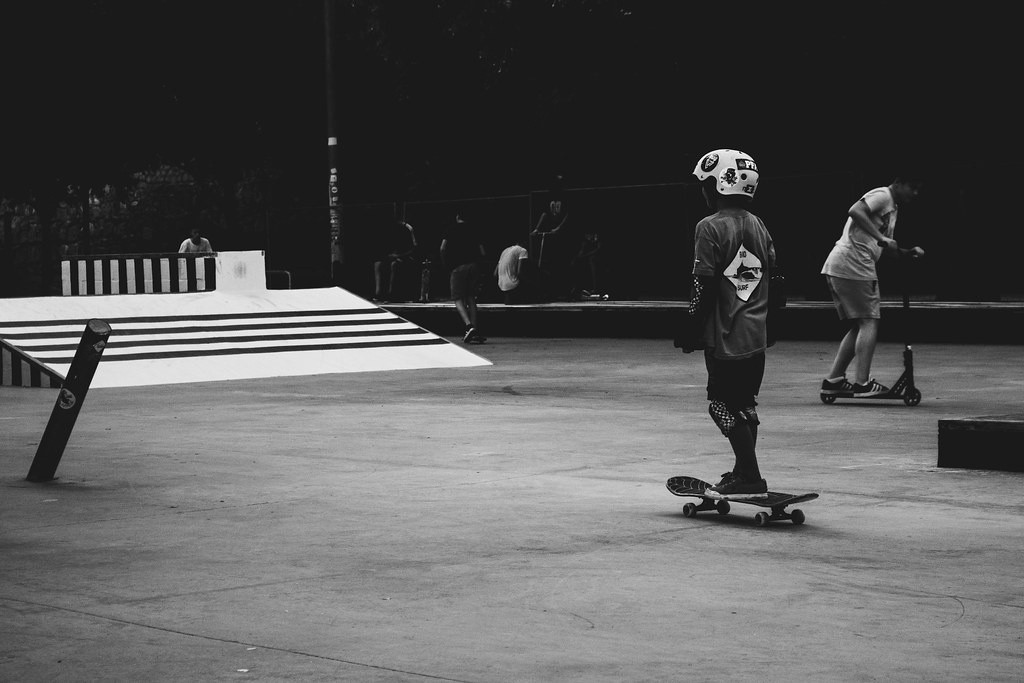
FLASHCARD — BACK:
[439,205,487,344]
[667,148,777,500]
[178,227,213,254]
[372,209,417,304]
[820,172,924,398]
[492,244,528,297]
[577,230,601,291]
[532,198,569,267]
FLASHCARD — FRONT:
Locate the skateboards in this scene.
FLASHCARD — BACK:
[419,258,433,303]
[463,330,489,347]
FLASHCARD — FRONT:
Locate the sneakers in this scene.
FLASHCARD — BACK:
[463,323,483,344]
[704,471,769,500]
[820,373,854,394]
[853,378,890,398]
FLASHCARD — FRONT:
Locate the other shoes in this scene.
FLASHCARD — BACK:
[582,288,596,295]
[373,296,381,301]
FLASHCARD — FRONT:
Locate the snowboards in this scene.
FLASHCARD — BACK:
[665,475,819,529]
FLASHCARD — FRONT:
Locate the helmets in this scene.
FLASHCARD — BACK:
[692,148,760,200]
